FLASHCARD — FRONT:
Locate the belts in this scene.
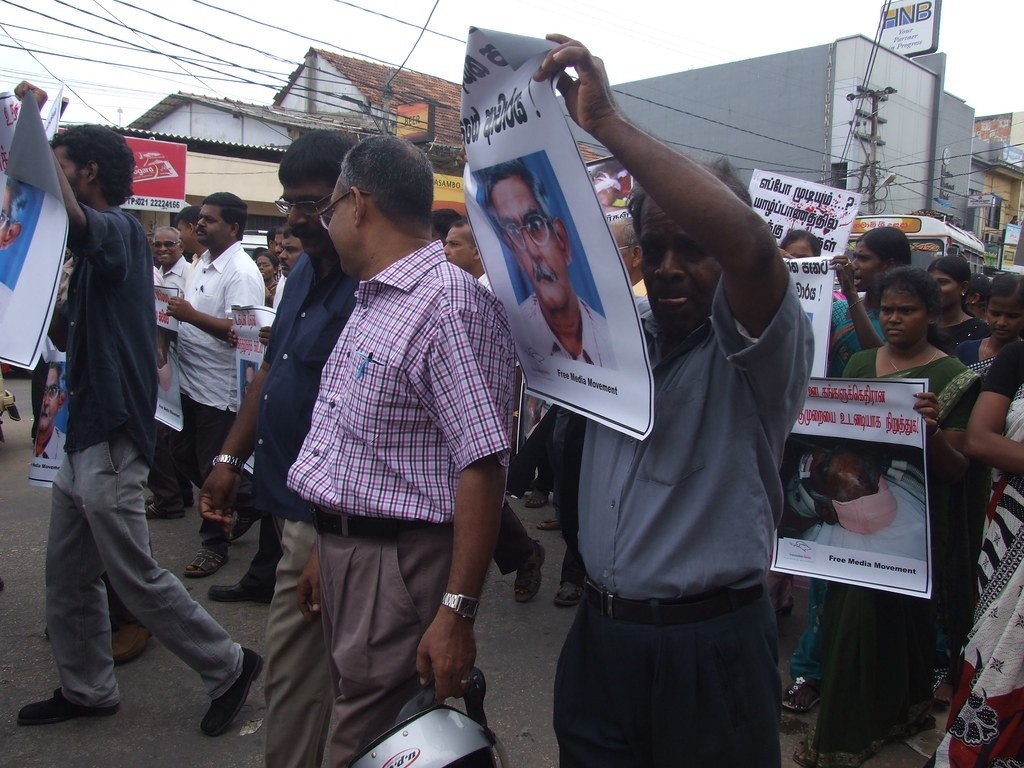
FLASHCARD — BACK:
[309,503,434,538]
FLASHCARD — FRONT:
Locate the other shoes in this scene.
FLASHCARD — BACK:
[110,621,153,661]
[538,516,560,529]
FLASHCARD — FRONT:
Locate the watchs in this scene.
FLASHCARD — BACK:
[442,589,480,619]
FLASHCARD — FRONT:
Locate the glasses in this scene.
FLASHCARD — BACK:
[503,213,551,249]
[153,239,180,249]
[275,194,335,216]
[317,189,371,228]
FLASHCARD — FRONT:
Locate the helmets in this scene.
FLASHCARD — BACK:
[345,705,498,768]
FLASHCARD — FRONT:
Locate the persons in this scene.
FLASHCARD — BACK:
[199,130,359,768]
[14,83,262,733]
[767,226,1024,768]
[778,433,898,539]
[34,365,67,459]
[283,135,517,768]
[532,32,814,768]
[479,158,614,368]
[1010,215,1018,224]
[37,192,646,673]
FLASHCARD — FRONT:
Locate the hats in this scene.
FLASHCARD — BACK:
[832,472,898,534]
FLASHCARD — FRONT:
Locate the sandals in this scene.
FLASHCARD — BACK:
[229,507,264,539]
[524,497,548,507]
[183,548,228,575]
[555,582,581,604]
[514,538,544,600]
[782,676,821,710]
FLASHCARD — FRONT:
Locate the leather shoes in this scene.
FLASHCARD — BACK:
[17,688,120,726]
[200,647,264,734]
[209,583,269,603]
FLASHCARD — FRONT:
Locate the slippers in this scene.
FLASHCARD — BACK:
[146,504,186,520]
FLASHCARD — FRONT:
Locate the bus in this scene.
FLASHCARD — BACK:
[834,213,988,292]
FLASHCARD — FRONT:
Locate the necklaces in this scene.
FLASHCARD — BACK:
[889,348,937,372]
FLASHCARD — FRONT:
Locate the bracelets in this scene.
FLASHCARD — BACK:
[212,454,244,471]
[848,299,862,310]
